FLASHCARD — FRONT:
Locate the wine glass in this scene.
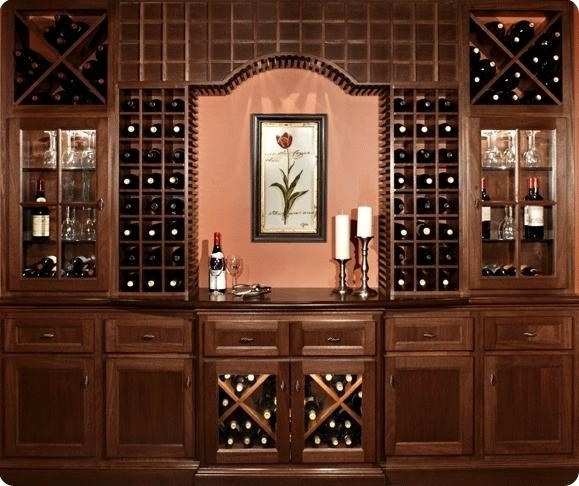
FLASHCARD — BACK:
[481,129,501,169]
[499,204,522,242]
[225,254,244,291]
[522,129,542,167]
[42,129,95,168]
[60,204,94,240]
[207,255,223,298]
[500,130,516,170]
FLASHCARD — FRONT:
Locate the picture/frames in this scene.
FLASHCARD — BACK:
[250,112,328,242]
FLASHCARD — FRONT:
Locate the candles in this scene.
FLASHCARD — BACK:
[356,206,373,237]
[334,214,351,260]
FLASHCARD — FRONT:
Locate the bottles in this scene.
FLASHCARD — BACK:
[22,255,57,278]
[13,14,108,103]
[208,231,228,294]
[483,265,543,278]
[469,20,561,103]
[210,372,364,449]
[61,256,97,279]
[478,177,492,239]
[120,96,183,293]
[521,174,546,240]
[30,178,51,241]
[392,95,457,290]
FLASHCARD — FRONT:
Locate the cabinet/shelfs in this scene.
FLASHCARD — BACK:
[383,308,476,479]
[1,306,102,478]
[115,79,195,298]
[466,4,570,108]
[196,310,381,482]
[387,81,466,300]
[10,7,114,110]
[7,110,114,297]
[477,309,578,480]
[102,307,198,478]
[466,107,571,298]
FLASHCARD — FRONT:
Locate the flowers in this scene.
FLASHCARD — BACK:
[268,132,310,225]
